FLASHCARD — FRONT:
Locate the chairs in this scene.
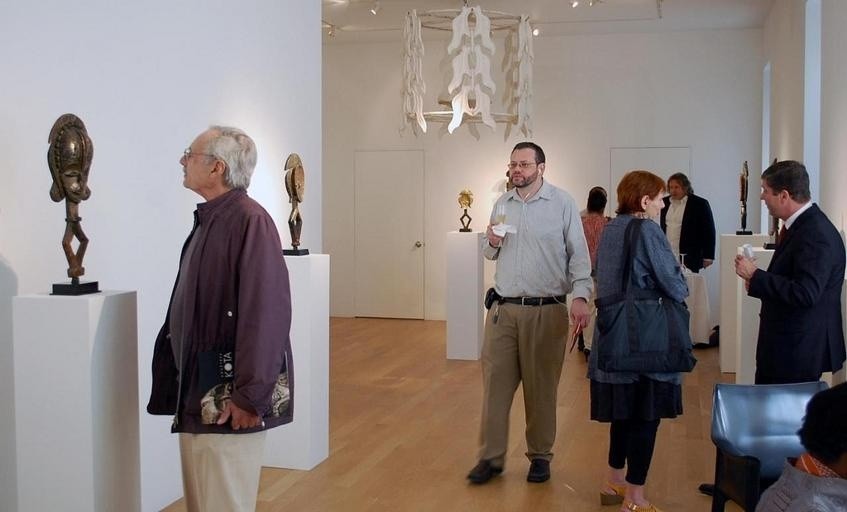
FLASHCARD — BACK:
[696,380,833,512]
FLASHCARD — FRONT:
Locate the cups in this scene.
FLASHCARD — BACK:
[736,245,753,259]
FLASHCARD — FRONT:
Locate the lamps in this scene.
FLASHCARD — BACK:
[404,0,535,138]
[368,1,382,16]
[322,19,341,38]
[531,25,540,37]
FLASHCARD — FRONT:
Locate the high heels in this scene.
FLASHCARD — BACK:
[583,348,591,363]
[619,499,661,512]
[600,480,627,505]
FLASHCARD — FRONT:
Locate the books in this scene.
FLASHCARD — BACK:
[197,344,290,434]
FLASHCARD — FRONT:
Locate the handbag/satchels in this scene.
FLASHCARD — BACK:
[593,292,697,373]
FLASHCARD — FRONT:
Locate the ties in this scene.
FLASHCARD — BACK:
[778,224,787,242]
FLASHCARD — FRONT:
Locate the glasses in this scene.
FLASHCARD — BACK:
[506,162,537,168]
[182,148,215,159]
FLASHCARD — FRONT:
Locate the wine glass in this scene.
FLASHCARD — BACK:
[679,254,686,274]
[494,204,510,225]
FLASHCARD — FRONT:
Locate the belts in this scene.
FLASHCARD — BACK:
[495,296,566,306]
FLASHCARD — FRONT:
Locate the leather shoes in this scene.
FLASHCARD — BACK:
[699,483,715,496]
[527,459,550,482]
[466,459,502,484]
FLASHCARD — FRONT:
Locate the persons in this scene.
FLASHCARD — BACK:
[145,123,295,511]
[578,187,612,221]
[587,168,697,511]
[696,158,845,500]
[574,184,611,362]
[658,170,716,350]
[752,380,845,510]
[465,139,596,488]
